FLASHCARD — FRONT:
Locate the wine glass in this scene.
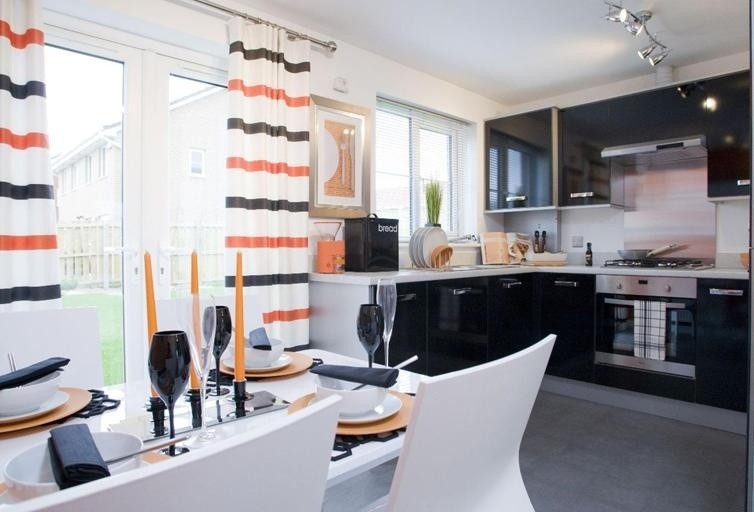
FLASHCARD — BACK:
[356,304,385,367]
[181,294,217,442]
[375,275,399,368]
[203,305,232,398]
[146,330,191,459]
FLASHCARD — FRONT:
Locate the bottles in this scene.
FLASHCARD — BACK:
[584,243,593,265]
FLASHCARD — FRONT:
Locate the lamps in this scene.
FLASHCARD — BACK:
[604,0,671,66]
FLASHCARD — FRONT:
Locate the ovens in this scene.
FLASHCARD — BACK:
[594,275,696,403]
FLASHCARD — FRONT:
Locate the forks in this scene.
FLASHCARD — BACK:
[339,128,348,187]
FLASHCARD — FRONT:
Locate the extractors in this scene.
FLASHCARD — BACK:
[600,134,707,168]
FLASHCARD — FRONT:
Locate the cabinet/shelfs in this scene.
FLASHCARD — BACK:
[485,70,752,213]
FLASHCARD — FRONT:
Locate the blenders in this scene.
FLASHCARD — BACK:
[313,221,347,274]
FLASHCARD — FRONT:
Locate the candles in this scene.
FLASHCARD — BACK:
[191,249,199,389]
[144,251,158,397]
[235,249,245,380]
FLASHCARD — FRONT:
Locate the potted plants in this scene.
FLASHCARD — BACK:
[425,179,442,227]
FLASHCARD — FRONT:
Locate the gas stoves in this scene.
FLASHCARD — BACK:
[600,257,713,269]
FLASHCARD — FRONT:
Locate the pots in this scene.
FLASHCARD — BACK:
[616,244,675,258]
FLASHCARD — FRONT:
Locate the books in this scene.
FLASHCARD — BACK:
[479,230,534,266]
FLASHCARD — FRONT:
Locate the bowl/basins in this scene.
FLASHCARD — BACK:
[228,337,284,366]
[313,371,388,416]
[3,430,142,494]
[524,251,568,261]
[0,372,60,413]
[432,247,453,267]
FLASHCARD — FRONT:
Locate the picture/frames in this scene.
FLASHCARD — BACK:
[309,93,372,219]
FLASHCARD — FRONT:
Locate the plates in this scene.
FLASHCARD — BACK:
[408,226,448,268]
[323,127,339,183]
[1,390,70,424]
[521,261,568,266]
[0,387,92,432]
[222,355,291,372]
[288,389,415,436]
[0,461,151,510]
[308,392,402,423]
[217,351,313,377]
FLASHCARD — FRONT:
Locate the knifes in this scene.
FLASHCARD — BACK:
[349,130,355,192]
